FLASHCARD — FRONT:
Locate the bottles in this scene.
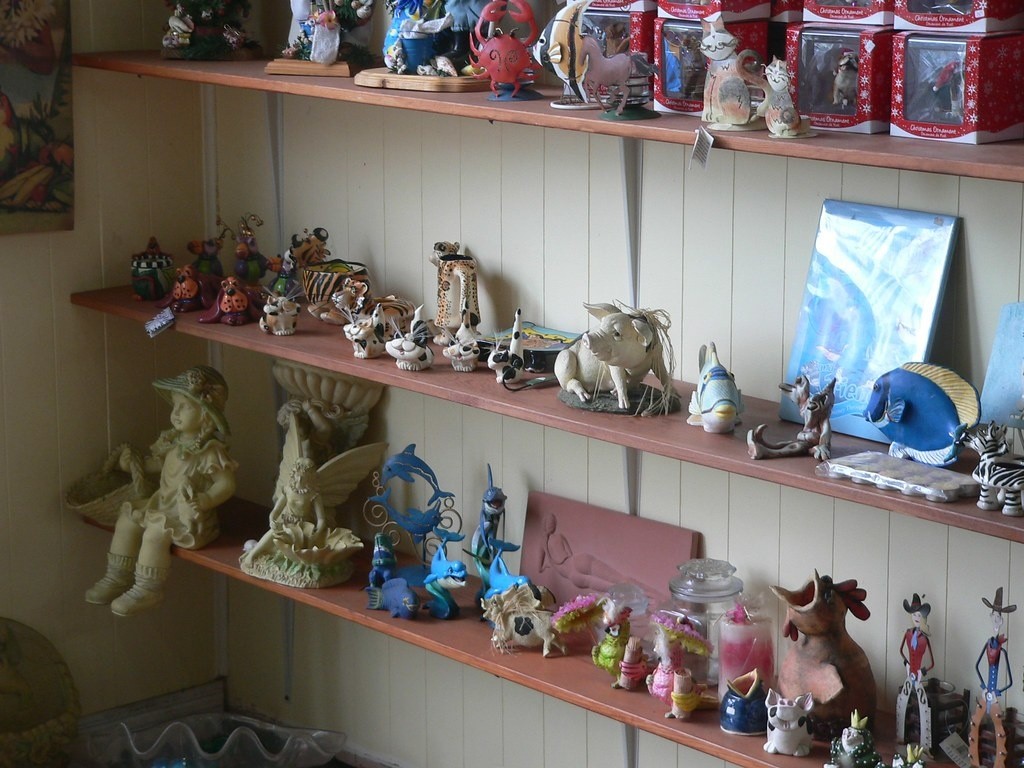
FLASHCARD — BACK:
[667,557,745,687]
[595,582,659,669]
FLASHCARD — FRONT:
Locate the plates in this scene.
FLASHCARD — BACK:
[85,711,347,768]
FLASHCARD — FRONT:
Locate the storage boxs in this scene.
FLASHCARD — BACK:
[567,0,1024,145]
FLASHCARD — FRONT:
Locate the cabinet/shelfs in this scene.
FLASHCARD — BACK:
[66,50,1024,768]
